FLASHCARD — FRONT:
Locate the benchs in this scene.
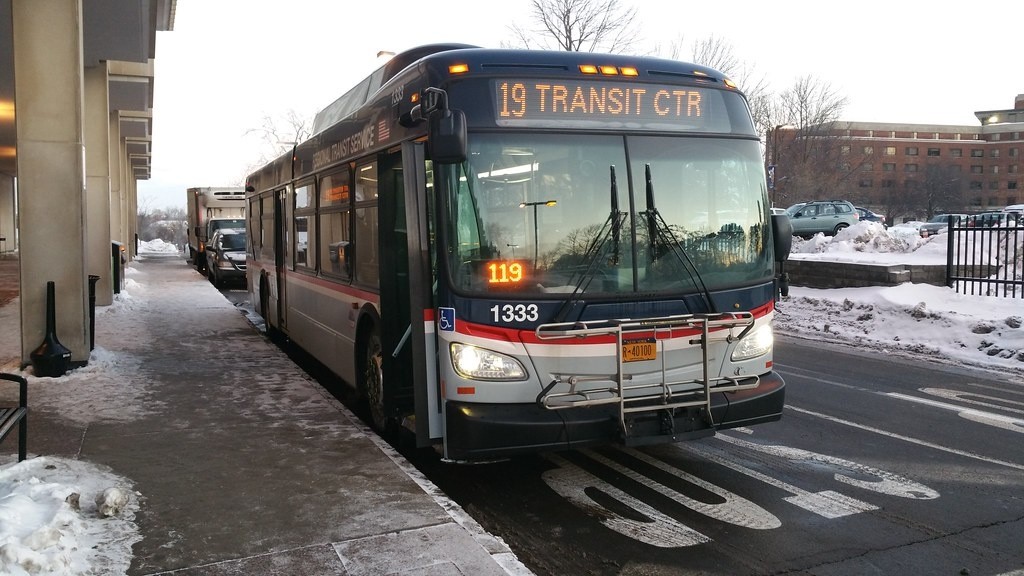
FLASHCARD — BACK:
[0,373,28,461]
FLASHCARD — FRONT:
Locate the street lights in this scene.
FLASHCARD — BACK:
[519,201,556,273]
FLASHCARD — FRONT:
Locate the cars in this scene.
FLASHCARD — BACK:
[957,203,1024,228]
[855,206,886,225]
[919,214,969,238]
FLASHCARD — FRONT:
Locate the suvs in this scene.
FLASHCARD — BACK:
[205,227,247,289]
[782,199,860,238]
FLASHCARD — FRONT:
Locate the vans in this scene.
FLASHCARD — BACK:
[769,209,785,216]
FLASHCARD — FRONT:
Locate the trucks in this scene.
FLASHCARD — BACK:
[187,186,245,271]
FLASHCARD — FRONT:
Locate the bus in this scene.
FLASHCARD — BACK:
[244,43,794,465]
